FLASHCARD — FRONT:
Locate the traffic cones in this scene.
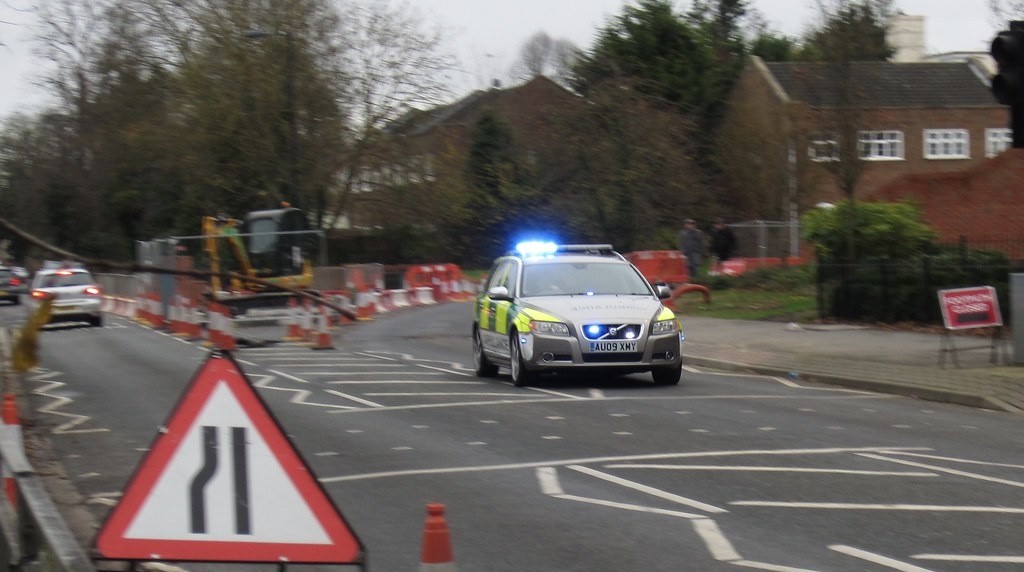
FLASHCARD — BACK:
[128,288,240,356]
[300,297,314,345]
[312,298,335,351]
[0,392,28,507]
[329,262,490,324]
[283,296,303,342]
[420,505,457,571]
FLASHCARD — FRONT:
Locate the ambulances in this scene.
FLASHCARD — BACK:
[471,243,683,388]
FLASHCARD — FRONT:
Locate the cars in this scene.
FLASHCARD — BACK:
[27,267,102,329]
[0,265,23,307]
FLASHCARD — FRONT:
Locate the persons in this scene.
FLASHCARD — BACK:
[710,220,738,262]
[678,218,710,281]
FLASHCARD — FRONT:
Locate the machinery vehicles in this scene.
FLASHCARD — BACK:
[197,213,315,304]
